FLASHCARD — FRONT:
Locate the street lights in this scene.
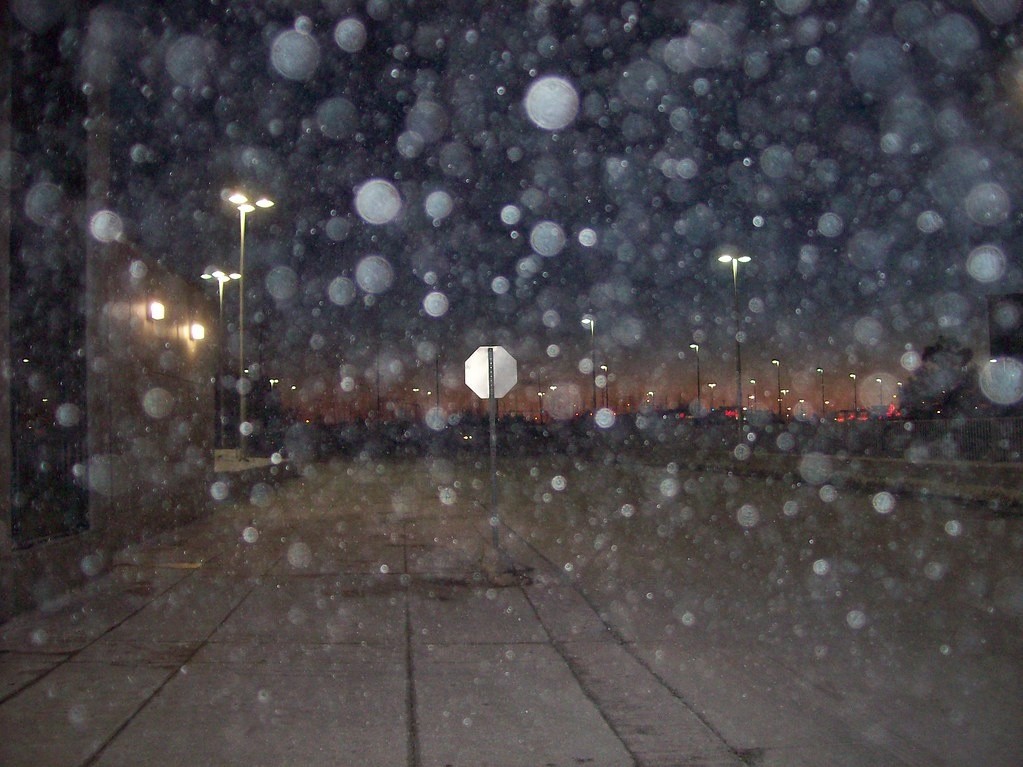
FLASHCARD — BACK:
[201,262,240,452]
[876,378,882,407]
[719,251,751,478]
[772,360,781,415]
[581,317,600,453]
[817,368,825,416]
[850,374,857,409]
[601,365,609,408]
[230,189,273,464]
[689,343,700,417]
